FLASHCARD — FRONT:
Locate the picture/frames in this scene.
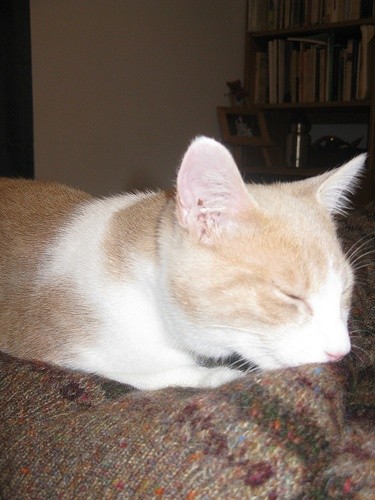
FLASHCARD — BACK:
[215,104,276,147]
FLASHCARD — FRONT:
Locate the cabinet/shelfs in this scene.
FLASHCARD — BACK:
[231,0,375,181]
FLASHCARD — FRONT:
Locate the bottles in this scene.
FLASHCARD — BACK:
[284,109,310,167]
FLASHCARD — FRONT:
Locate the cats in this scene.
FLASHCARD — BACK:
[0,135,369,392]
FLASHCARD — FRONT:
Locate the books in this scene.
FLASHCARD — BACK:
[240,0,375,103]
